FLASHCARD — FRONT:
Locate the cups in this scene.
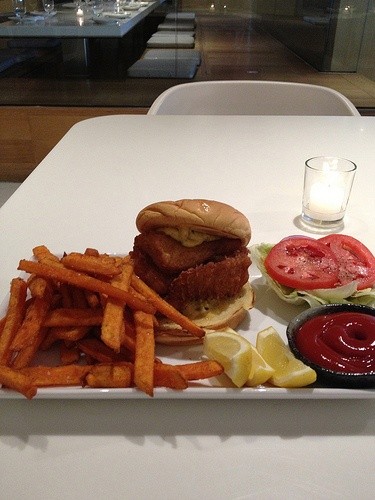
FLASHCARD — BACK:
[299,156,357,230]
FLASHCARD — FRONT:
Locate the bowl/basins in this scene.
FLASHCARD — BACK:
[287,303,375,388]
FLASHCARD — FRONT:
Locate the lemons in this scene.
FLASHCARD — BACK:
[257,327,316,387]
[204,326,275,387]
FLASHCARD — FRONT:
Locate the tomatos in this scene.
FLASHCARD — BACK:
[317,234,375,290]
[264,238,339,289]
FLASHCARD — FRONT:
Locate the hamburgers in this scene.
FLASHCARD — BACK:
[129,199,256,346]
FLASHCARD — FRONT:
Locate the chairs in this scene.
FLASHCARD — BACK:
[148,79,360,117]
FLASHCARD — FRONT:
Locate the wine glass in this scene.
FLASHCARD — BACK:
[74,0,135,17]
[13,0,54,25]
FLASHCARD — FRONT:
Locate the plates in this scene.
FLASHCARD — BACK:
[8,10,56,21]
[103,1,149,17]
[62,1,94,8]
[0,251,375,400]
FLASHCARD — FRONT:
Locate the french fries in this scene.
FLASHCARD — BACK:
[0,245,224,401]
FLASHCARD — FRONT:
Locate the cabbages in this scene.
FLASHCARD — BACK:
[247,243,375,307]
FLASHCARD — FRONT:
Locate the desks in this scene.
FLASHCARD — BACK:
[0,114,375,500]
